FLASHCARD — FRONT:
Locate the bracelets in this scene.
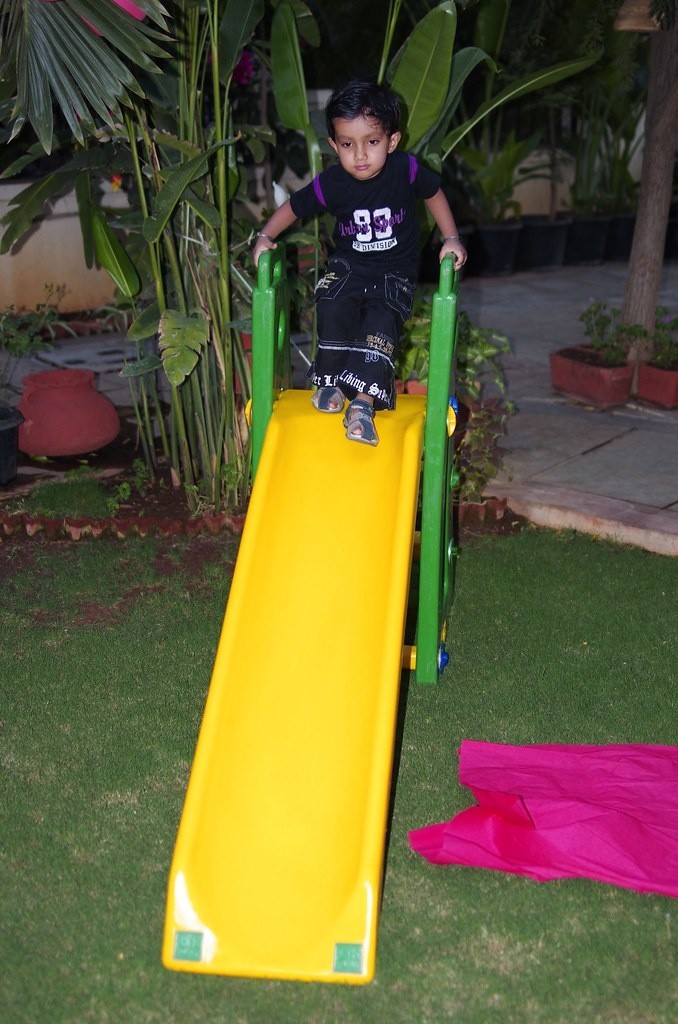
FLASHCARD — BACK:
[442,236,461,243]
[255,231,275,244]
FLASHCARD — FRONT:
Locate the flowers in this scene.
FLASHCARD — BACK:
[640,307,678,368]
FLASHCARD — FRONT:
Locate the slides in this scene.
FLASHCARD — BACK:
[160,388,458,987]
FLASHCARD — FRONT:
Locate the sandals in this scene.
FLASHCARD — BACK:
[342,398,380,446]
[310,386,345,413]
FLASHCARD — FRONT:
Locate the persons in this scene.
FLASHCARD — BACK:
[250,81,467,448]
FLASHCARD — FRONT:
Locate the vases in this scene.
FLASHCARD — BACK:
[638,364,678,410]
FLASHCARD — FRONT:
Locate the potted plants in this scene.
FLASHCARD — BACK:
[549,301,646,407]
[0,282,69,487]
[419,0,678,285]
